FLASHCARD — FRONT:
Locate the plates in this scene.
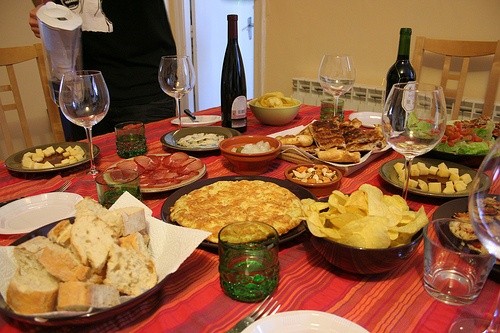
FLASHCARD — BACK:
[103,115,242,193]
[0,217,163,325]
[160,176,318,251]
[239,309,370,333]
[0,142,100,234]
[266,110,500,282]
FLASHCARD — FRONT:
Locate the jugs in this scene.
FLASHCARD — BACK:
[35,1,82,82]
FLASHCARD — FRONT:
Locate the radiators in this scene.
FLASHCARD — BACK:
[291,77,500,122]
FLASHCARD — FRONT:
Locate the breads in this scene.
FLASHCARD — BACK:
[7,196,158,313]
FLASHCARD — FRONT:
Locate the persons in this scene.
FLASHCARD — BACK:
[28,0,179,143]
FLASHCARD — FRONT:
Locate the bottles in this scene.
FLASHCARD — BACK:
[221,14,247,134]
[385,27,416,101]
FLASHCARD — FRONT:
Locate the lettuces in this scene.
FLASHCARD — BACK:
[406,114,495,154]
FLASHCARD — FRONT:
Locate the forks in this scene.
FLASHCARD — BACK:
[226,295,281,333]
[0,179,72,207]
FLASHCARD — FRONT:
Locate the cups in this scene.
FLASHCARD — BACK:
[114,121,146,158]
[320,99,344,122]
[423,219,496,305]
[95,169,143,209]
[219,220,280,303]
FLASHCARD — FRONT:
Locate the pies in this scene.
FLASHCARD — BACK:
[276,118,391,162]
[170,180,303,243]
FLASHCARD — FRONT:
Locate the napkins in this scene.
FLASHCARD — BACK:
[0,190,212,319]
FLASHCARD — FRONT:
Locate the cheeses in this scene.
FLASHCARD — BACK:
[22,146,85,168]
[394,163,472,194]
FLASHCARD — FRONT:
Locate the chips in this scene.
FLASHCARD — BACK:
[254,92,294,108]
[300,183,429,249]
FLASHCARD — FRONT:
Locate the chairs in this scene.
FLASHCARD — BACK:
[412,36,500,121]
[0,44,66,160]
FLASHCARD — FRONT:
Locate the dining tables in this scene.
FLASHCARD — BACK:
[0,98,500,333]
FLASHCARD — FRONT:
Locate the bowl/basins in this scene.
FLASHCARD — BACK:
[249,98,302,126]
[219,135,342,198]
[302,197,423,275]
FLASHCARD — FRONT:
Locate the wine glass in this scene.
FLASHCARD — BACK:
[58,68,110,181]
[319,54,356,120]
[157,54,193,130]
[382,81,448,199]
[468,133,500,333]
